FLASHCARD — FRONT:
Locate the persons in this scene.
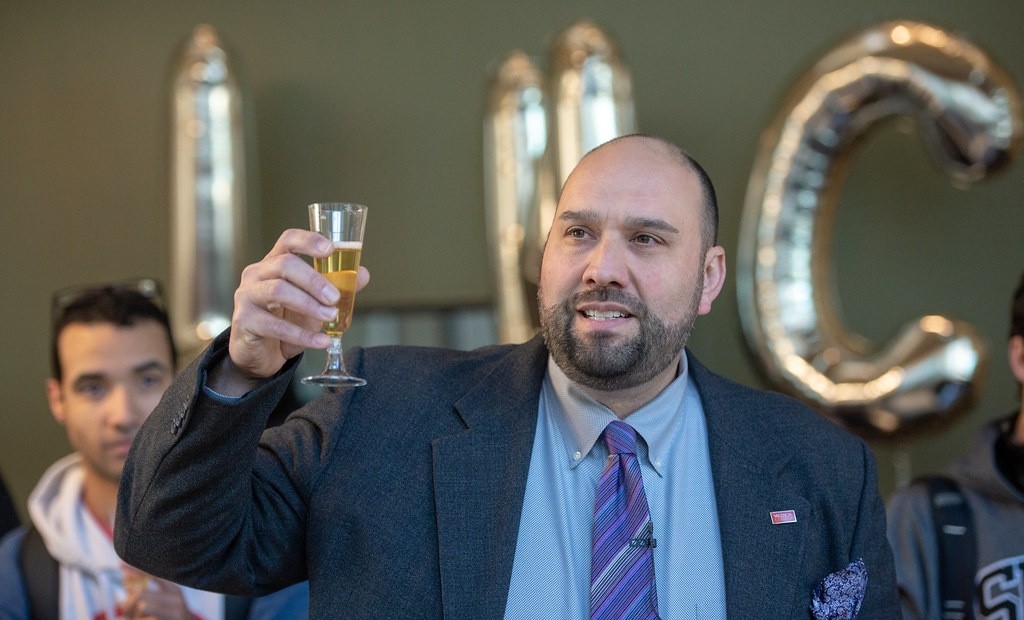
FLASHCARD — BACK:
[0,286,308,620]
[885,272,1024,620]
[114,133,903,620]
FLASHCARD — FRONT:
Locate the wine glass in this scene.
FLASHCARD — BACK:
[301,203,370,388]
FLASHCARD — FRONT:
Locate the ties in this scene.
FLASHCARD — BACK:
[589,420,663,620]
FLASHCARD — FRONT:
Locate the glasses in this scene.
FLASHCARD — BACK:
[48,276,169,320]
